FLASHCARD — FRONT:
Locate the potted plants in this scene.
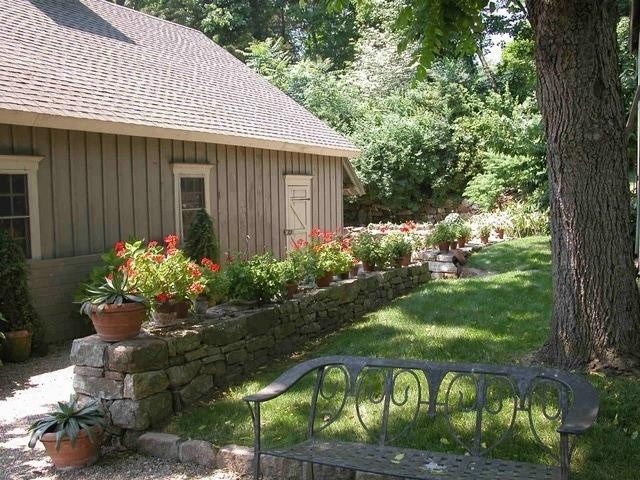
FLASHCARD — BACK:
[335,242,349,278]
[142,238,187,326]
[189,258,221,313]
[347,240,361,277]
[79,241,147,338]
[280,267,297,300]
[380,235,412,268]
[176,268,193,316]
[359,235,377,272]
[425,225,505,252]
[313,245,332,287]
[27,395,111,470]
[0,231,43,364]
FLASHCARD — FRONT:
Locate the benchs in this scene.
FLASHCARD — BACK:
[242,352,600,480]
